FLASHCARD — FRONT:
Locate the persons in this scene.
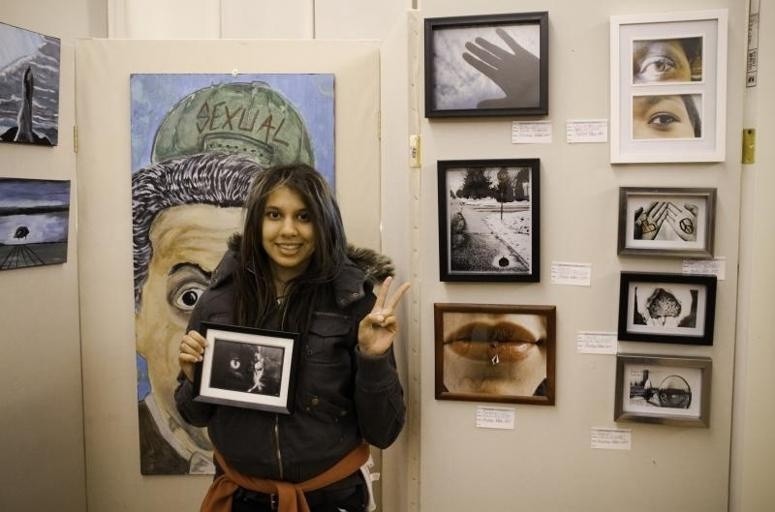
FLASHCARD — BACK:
[463,28,541,108]
[131,155,265,475]
[633,200,699,242]
[633,94,701,139]
[174,162,412,512]
[633,38,700,82]
[443,312,548,396]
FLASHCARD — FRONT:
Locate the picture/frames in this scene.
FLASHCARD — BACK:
[612,352,713,429]
[617,270,716,347]
[433,303,556,406]
[191,319,302,417]
[617,185,717,260]
[438,157,541,284]
[611,6,726,164]
[424,11,548,119]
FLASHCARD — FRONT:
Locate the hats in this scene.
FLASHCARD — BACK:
[149,79,314,172]
